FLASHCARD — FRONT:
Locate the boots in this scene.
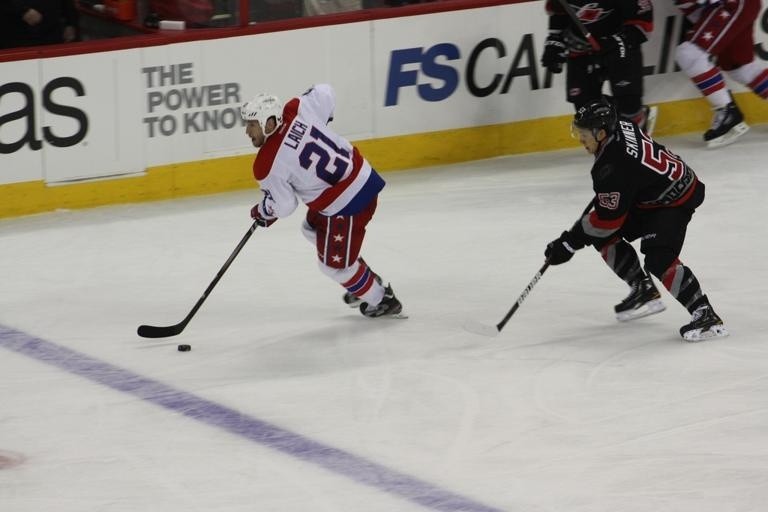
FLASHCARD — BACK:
[679,293,724,338]
[613,265,663,314]
[702,89,745,142]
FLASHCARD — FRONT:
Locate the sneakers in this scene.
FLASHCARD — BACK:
[360,282,403,319]
[620,103,651,133]
[342,268,383,305]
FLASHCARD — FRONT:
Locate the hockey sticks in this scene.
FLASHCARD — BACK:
[452,196,596,336]
[138,222,258,338]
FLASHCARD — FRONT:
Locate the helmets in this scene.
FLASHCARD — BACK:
[239,91,285,139]
[568,99,618,145]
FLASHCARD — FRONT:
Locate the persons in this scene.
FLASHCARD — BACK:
[666,0,768,140]
[240,83,403,319]
[543,0,658,139]
[540,98,726,342]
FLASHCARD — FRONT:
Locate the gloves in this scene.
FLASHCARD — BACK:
[591,32,633,70]
[544,230,581,266]
[249,195,278,228]
[542,35,571,76]
